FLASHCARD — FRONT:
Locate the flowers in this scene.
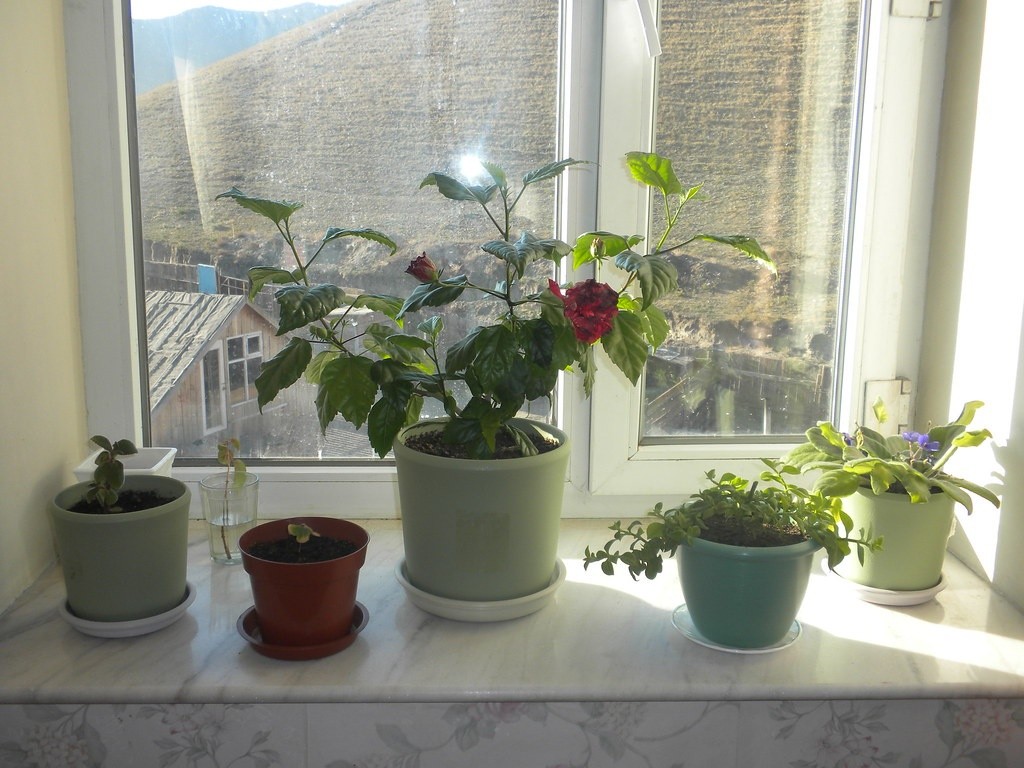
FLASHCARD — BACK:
[211,151,779,462]
[782,392,1000,535]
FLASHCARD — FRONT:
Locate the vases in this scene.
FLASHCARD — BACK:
[391,417,569,602]
[200,470,259,566]
[836,485,956,589]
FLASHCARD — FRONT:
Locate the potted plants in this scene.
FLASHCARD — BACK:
[51,434,190,620]
[583,468,885,649]
[238,516,372,645]
